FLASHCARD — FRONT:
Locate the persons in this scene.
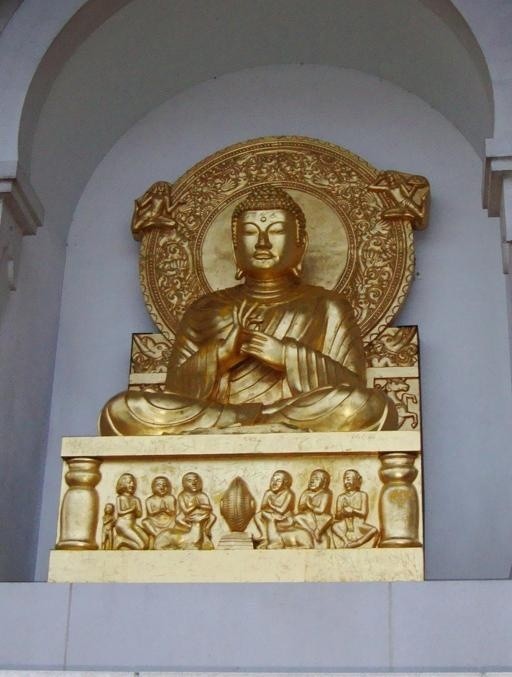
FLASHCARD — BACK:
[331,470,377,547]
[100,503,115,550]
[134,182,185,232]
[176,472,218,535]
[114,473,150,549]
[294,469,332,541]
[253,469,294,549]
[142,476,176,538]
[368,169,426,219]
[98,185,399,437]
